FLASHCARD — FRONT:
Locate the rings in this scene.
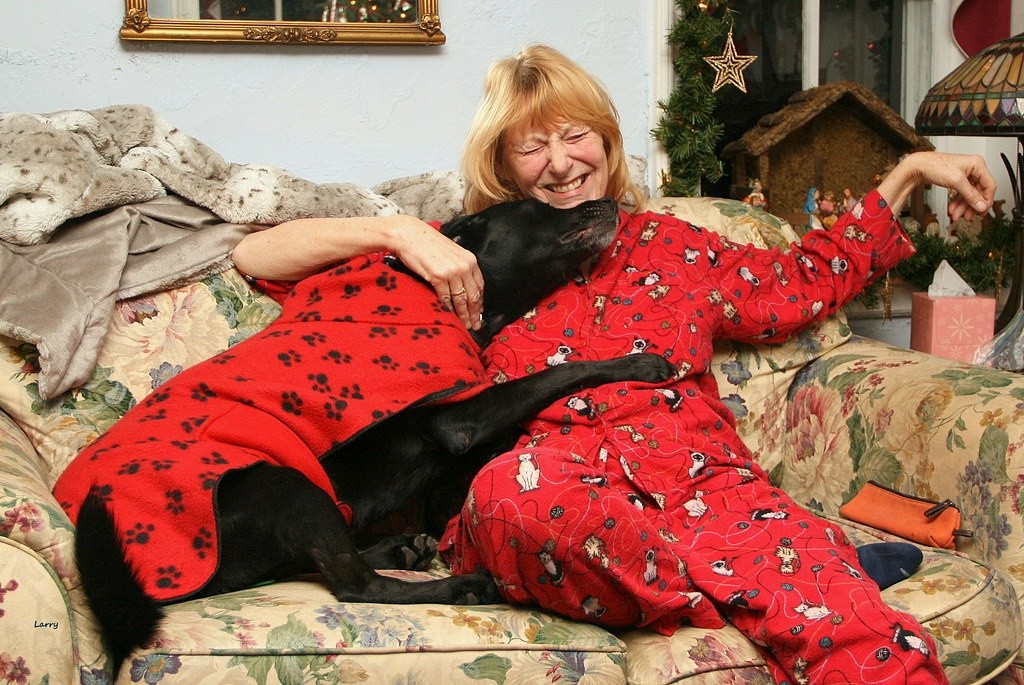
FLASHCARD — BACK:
[452,288,466,295]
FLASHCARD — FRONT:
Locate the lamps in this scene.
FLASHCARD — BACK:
[913,31,1024,334]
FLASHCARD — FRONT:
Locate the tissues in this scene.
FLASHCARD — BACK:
[910,259,995,361]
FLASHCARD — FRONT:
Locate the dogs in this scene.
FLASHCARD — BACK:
[74,195,678,664]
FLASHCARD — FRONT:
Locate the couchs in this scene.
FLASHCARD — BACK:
[0,197,1023,685]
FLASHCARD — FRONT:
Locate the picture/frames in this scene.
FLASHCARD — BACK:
[119,0,447,45]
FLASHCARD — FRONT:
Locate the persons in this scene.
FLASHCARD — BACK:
[744,179,858,232]
[231,45,997,685]
